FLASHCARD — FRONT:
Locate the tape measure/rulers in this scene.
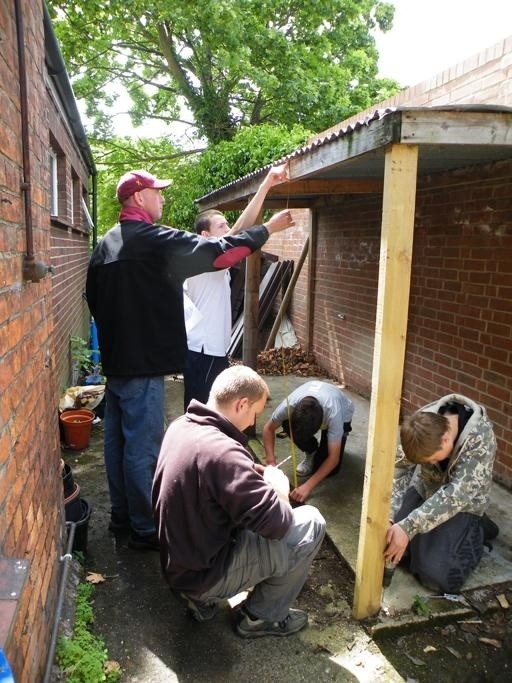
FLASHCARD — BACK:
[280,159,298,489]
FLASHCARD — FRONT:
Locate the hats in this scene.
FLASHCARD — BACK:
[116,170,172,203]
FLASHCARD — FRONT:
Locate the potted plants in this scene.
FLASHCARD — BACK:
[70,336,106,418]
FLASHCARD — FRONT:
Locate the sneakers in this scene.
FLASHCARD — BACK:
[236,607,308,639]
[179,591,218,623]
[481,512,499,539]
[109,510,133,528]
[128,532,162,551]
[296,450,317,476]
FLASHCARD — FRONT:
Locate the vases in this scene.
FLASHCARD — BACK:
[59,459,91,559]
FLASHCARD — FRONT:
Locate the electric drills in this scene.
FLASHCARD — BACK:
[382,558,397,603]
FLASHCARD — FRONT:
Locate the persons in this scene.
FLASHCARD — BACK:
[381,393,498,595]
[86,170,295,553]
[182,160,292,413]
[263,379,355,503]
[151,365,327,638]
